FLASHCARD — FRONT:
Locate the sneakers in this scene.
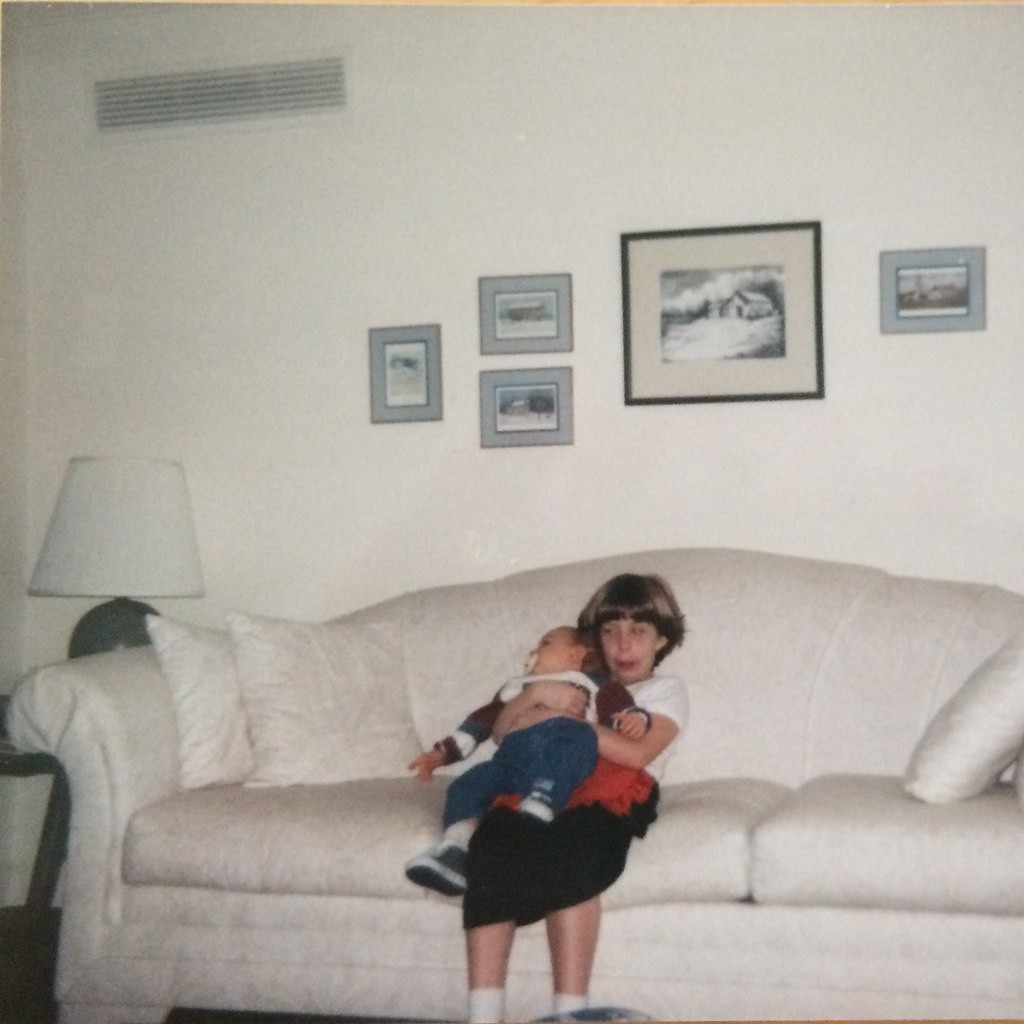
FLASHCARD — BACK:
[520,774,555,823]
[404,843,468,898]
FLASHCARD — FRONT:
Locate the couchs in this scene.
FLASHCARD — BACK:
[5,547,1024,1024]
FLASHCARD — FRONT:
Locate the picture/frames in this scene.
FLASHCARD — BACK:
[477,271,575,356]
[367,321,445,424]
[620,220,828,406]
[878,245,990,336]
[478,364,578,450]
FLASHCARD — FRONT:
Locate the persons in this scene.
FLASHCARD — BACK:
[465,572,691,1024]
[406,625,652,900]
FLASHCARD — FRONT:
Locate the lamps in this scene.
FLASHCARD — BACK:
[27,455,208,659]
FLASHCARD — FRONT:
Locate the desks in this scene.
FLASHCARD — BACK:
[0,692,71,1024]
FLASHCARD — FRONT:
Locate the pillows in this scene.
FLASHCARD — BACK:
[906,626,1024,805]
[145,611,256,793]
[224,610,424,790]
